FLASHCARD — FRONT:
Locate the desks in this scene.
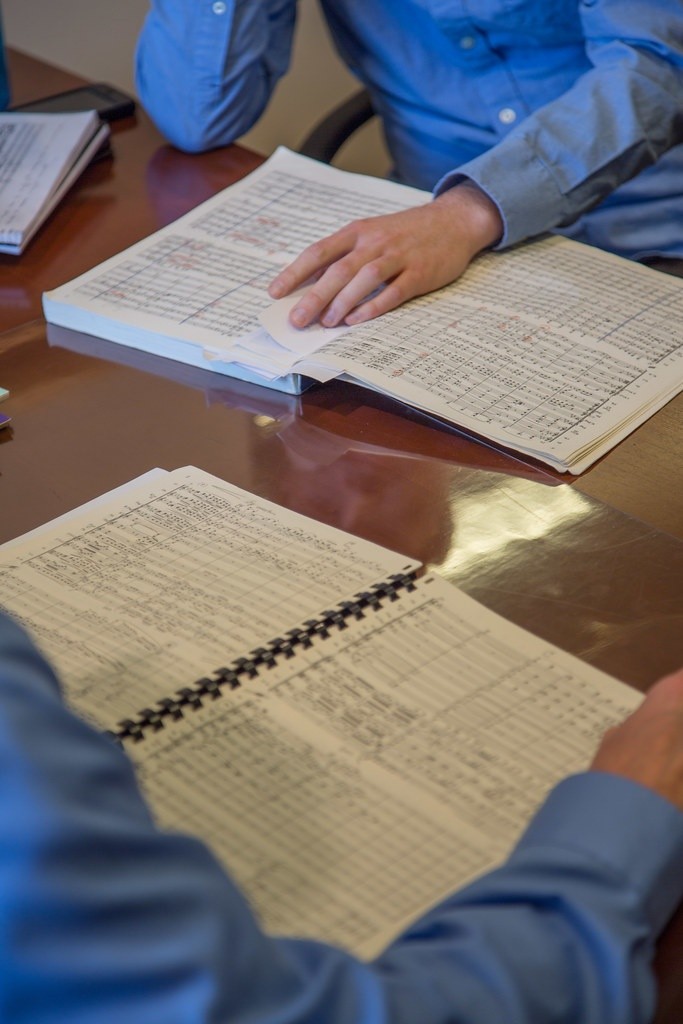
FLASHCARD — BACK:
[0,42,683,959]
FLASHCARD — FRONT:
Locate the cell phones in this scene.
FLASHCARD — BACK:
[7,83,137,125]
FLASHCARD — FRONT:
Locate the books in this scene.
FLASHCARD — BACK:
[40,143,683,475]
[0,462,649,957]
[0,109,113,255]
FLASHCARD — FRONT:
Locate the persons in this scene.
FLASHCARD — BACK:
[1,602,683,1024]
[131,0,683,330]
